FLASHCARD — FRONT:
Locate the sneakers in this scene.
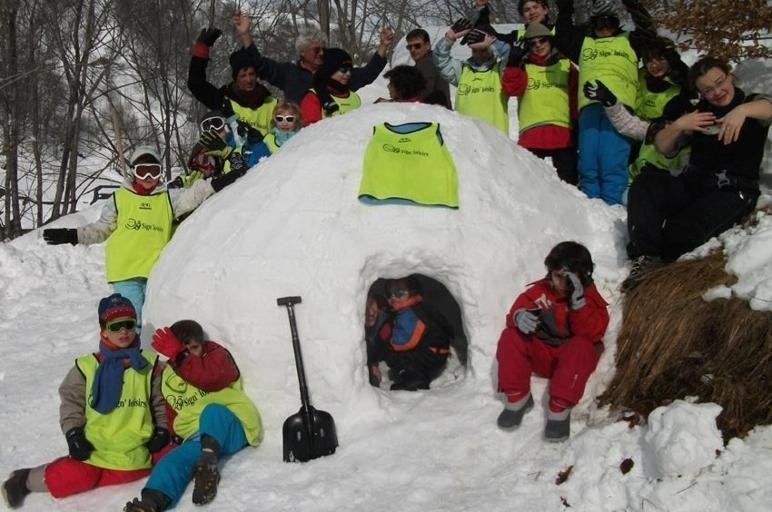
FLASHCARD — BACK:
[193,464,220,504]
[498,394,533,429]
[1,468,29,508]
[123,497,156,512]
[546,408,570,442]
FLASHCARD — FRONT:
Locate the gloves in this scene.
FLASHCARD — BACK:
[66,427,93,460]
[514,308,541,334]
[583,80,616,107]
[561,272,585,311]
[200,129,233,160]
[151,327,185,360]
[147,427,169,452]
[43,228,77,246]
[446,18,497,49]
[508,41,527,65]
[192,28,220,57]
[212,169,246,192]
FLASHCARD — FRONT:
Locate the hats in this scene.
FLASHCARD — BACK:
[98,293,136,323]
[130,146,160,168]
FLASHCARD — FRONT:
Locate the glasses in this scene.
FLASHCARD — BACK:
[406,44,421,49]
[106,318,136,333]
[338,63,353,72]
[134,163,161,179]
[200,116,227,133]
[530,39,547,46]
[314,47,324,52]
[385,289,407,298]
[274,115,296,123]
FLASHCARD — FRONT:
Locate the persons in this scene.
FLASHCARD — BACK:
[365,289,400,387]
[404,29,452,109]
[374,65,426,105]
[555,1,658,203]
[376,277,456,391]
[123,320,263,512]
[621,56,772,293]
[169,112,271,228]
[232,10,393,107]
[501,21,579,185]
[432,13,510,135]
[583,33,702,186]
[496,241,609,442]
[187,27,278,138]
[2,293,170,509]
[43,144,246,336]
[473,0,574,50]
[263,98,305,154]
[300,48,361,128]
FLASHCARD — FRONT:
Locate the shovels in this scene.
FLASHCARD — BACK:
[277,296,339,463]
